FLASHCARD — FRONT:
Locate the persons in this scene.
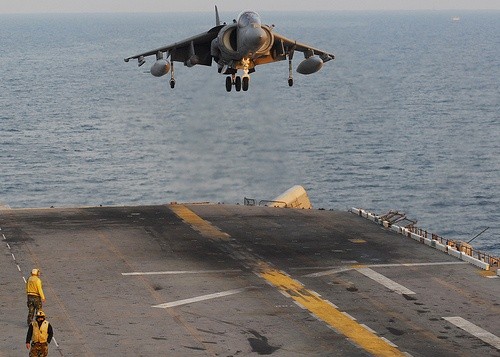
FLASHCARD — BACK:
[26,269,45,325]
[25,312,53,357]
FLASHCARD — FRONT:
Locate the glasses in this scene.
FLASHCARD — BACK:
[36,316,45,319]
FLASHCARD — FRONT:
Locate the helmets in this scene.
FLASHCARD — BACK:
[36,310,46,317]
[31,268,40,277]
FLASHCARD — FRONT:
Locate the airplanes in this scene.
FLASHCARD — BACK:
[125,5,334,92]
